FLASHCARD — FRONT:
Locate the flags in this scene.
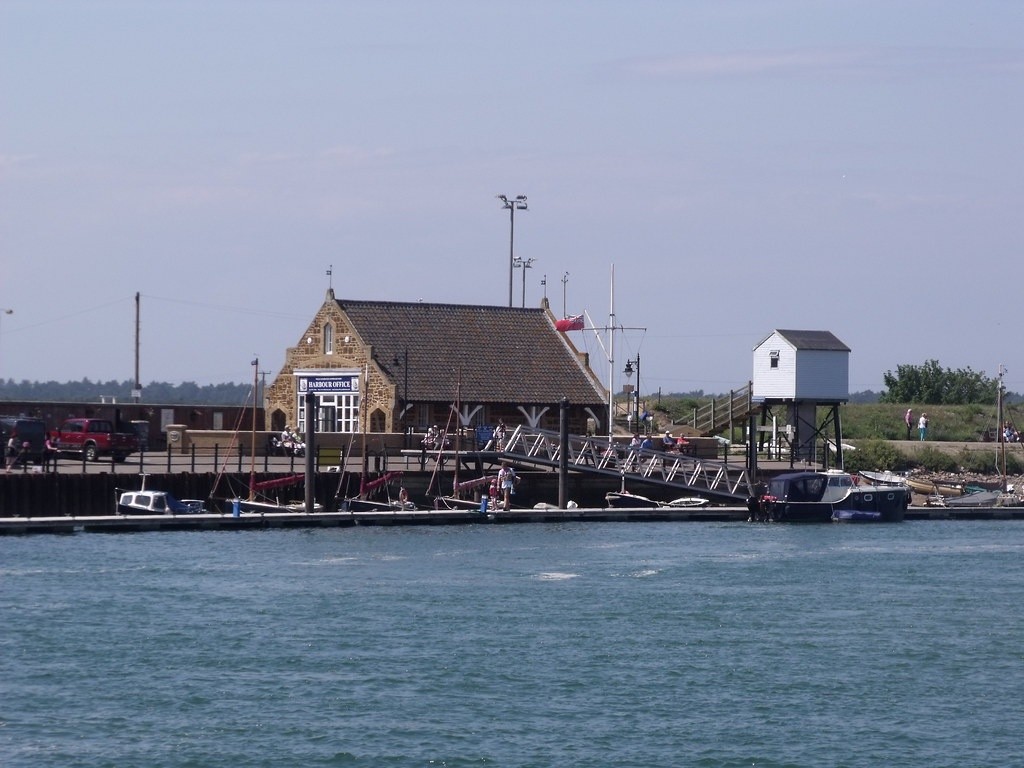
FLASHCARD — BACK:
[554,315,585,332]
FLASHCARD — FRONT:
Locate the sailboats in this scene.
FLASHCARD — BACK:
[208,359,325,514]
[334,362,419,511]
[423,364,579,510]
[114,472,206,515]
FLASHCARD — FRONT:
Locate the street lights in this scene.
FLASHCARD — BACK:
[390,344,407,455]
[623,352,639,436]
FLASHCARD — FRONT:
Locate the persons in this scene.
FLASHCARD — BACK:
[4,432,20,473]
[904,408,913,440]
[631,433,642,453]
[489,478,499,511]
[495,417,507,444]
[916,413,930,441]
[281,425,307,455]
[677,432,690,454]
[421,424,447,456]
[497,461,516,511]
[641,435,654,452]
[662,431,680,454]
[41,434,59,473]
[1003,420,1020,443]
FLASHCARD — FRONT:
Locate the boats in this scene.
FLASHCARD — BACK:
[745,470,912,523]
[606,468,710,508]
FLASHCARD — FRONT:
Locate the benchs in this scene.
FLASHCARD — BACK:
[660,438,696,457]
[268,434,307,456]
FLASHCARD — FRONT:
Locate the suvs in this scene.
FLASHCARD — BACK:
[0,414,49,464]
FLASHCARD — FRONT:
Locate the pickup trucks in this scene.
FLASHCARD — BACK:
[45,418,139,464]
